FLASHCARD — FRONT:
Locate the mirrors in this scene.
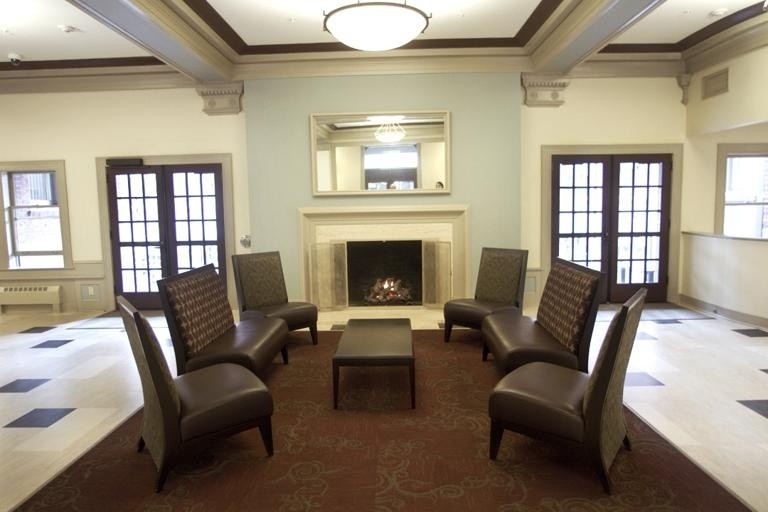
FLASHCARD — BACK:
[309,110,453,199]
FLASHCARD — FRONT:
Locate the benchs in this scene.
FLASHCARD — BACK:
[156,263,289,385]
[481,256,607,379]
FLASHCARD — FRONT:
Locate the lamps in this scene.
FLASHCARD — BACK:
[316,0,433,52]
[374,123,406,144]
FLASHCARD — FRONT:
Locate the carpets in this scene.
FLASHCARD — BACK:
[7,330,755,512]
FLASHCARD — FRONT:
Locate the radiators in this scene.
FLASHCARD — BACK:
[0,285,61,313]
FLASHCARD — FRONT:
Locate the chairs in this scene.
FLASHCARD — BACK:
[443,246,529,343]
[230,252,317,346]
[116,294,276,493]
[484,286,647,495]
